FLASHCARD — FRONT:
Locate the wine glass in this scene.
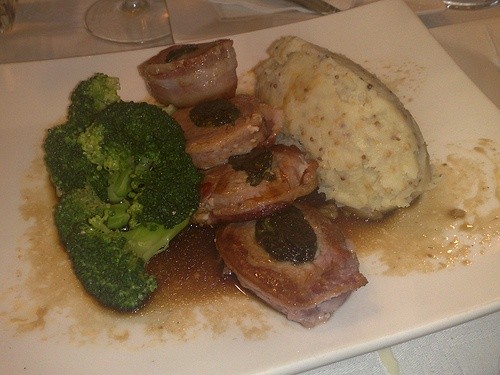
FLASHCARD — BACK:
[83,1,171,43]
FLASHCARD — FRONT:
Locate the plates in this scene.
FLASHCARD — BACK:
[0,0,500,375]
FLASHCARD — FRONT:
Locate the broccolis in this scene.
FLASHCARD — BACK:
[41,74,204,309]
